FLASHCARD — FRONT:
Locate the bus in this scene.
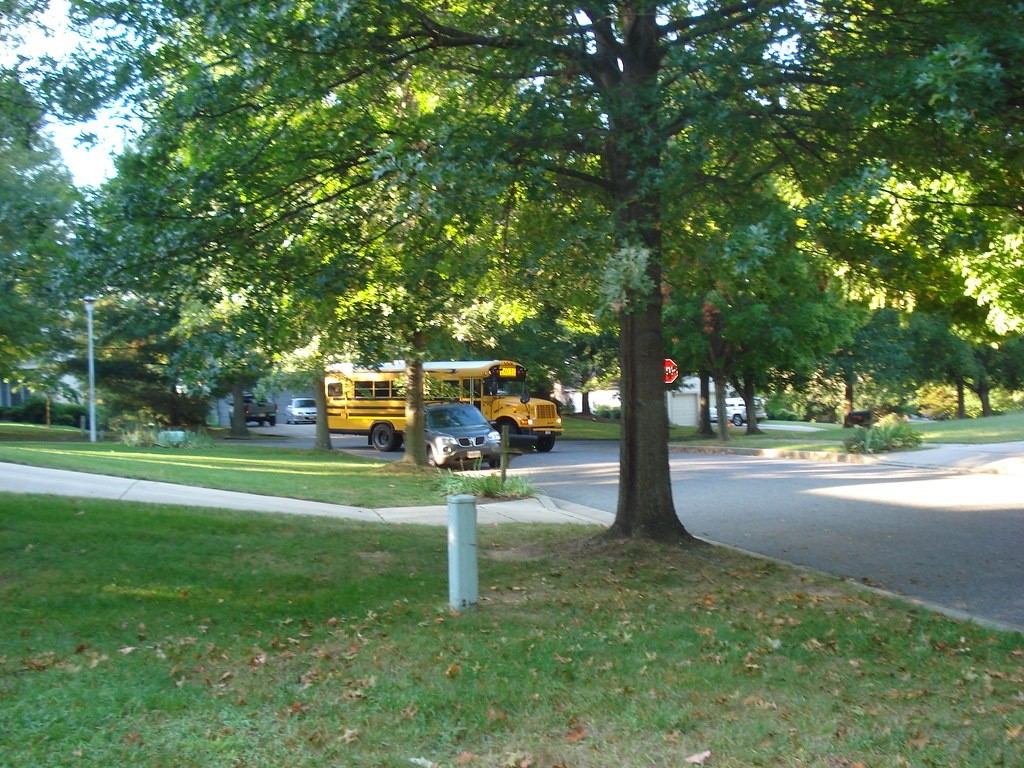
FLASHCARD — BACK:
[323,360,565,452]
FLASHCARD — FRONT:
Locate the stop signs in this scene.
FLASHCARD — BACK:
[664,359,678,384]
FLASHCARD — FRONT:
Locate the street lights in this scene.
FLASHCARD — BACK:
[84,294,99,446]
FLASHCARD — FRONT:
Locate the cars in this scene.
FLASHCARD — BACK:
[422,397,502,468]
[710,397,765,425]
[285,397,317,424]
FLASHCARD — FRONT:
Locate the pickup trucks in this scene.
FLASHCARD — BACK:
[225,392,279,426]
